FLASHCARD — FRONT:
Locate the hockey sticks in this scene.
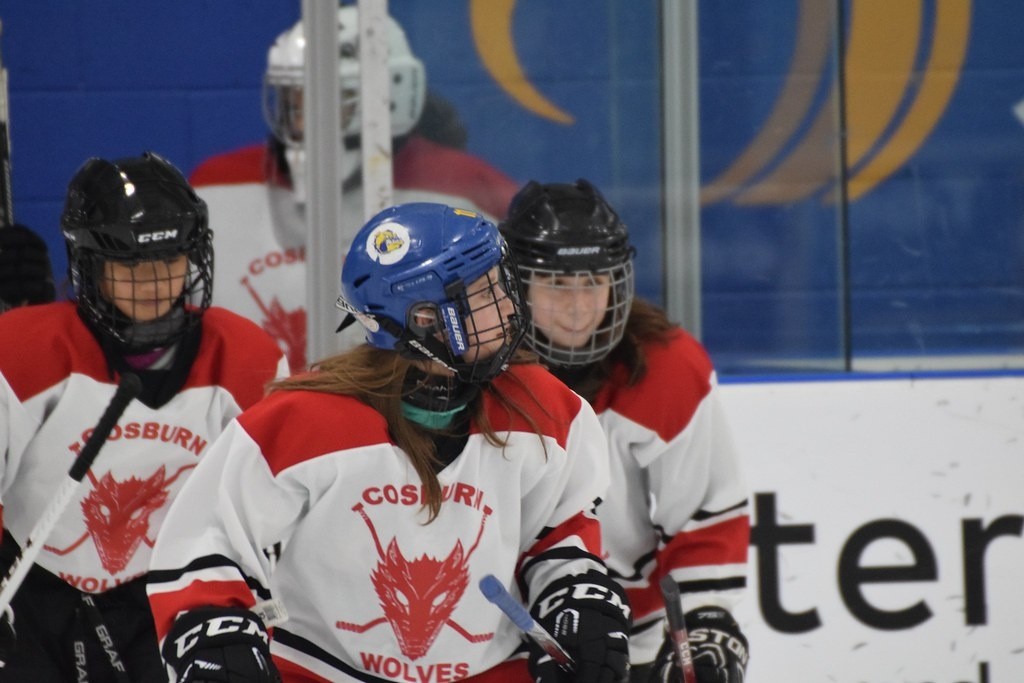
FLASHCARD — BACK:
[656,575,700,682]
[0,372,145,614]
[477,575,577,666]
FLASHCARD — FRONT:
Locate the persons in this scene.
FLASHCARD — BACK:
[498,177,750,683]
[0,155,291,683]
[146,203,634,683]
[187,4,522,379]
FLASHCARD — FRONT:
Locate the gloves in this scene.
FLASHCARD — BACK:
[162,606,282,683]
[0,225,56,306]
[651,606,749,683]
[526,569,633,683]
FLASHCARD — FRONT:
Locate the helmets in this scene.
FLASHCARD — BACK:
[335,202,530,384]
[497,178,638,365]
[60,151,215,353]
[262,5,426,202]
